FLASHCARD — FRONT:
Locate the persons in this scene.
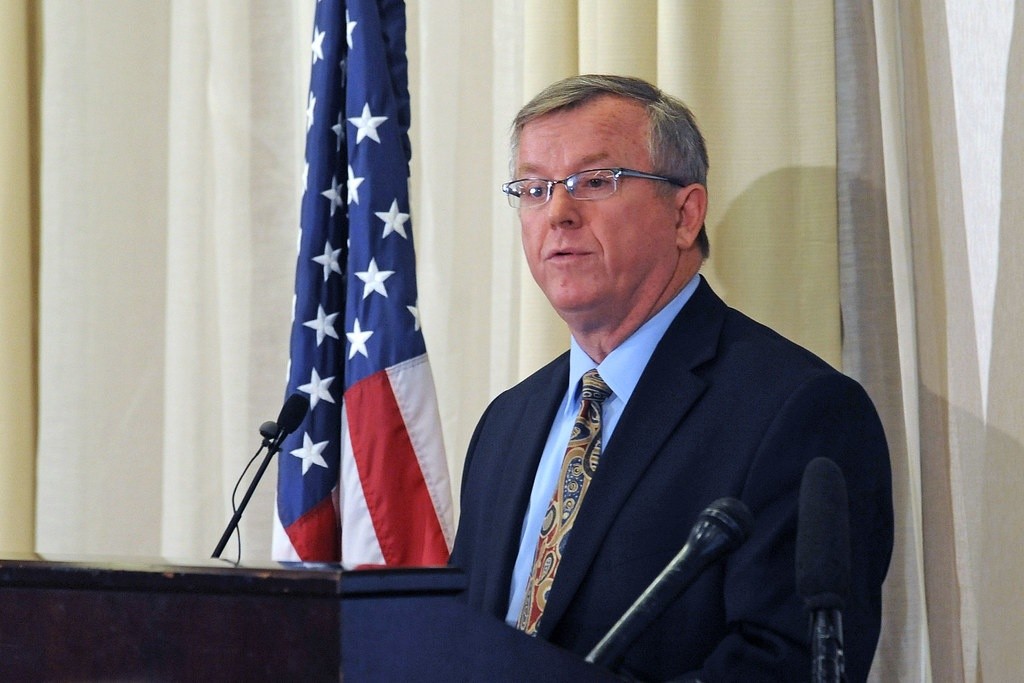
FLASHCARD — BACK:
[448,73,895,682]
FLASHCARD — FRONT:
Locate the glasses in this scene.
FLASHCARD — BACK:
[502,167,685,210]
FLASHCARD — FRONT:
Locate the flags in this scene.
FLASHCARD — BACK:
[276,0,457,572]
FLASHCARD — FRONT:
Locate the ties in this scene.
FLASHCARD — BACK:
[516,368,611,638]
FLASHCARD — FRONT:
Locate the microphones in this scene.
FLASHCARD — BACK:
[794,457,849,683]
[585,497,755,672]
[211,396,310,558]
